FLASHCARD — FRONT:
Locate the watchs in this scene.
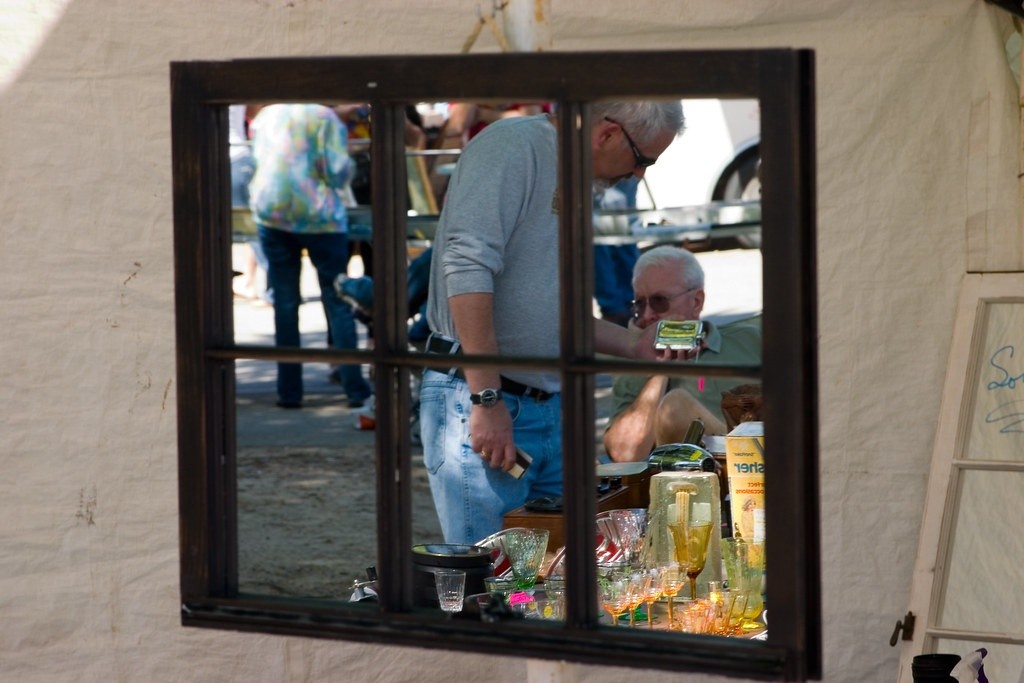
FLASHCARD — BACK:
[470,389,503,407]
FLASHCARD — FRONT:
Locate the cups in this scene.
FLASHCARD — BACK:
[709,578,724,592]
[466,592,503,620]
[435,569,466,612]
[484,576,519,605]
[536,600,556,619]
[611,508,659,571]
[674,597,717,635]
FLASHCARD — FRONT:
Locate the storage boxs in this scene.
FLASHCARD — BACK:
[726,420,764,574]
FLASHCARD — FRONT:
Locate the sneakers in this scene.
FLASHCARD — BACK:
[334,275,375,318]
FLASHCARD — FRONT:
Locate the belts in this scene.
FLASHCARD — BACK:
[428,338,558,401]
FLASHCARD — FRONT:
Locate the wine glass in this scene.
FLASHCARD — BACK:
[544,576,565,620]
[629,569,666,631]
[700,592,734,637]
[657,563,688,628]
[722,537,767,628]
[507,529,549,614]
[667,520,713,600]
[611,574,651,628]
[723,589,750,634]
[603,581,633,627]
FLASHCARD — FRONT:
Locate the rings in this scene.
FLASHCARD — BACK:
[481,451,489,457]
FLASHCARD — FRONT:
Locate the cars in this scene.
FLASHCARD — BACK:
[636,98,762,249]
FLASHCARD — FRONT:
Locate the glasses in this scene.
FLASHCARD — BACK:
[632,288,697,313]
[601,116,656,170]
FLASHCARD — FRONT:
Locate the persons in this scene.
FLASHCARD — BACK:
[245,95,763,547]
